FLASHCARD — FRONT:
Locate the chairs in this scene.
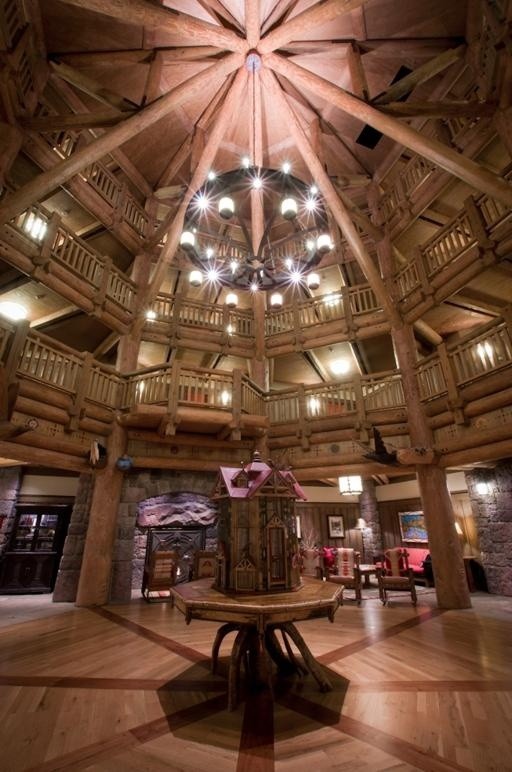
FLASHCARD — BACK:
[378,549,417,607]
[142,549,219,603]
[324,548,361,604]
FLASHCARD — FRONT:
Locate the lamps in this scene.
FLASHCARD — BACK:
[338,475,363,496]
[178,50,332,309]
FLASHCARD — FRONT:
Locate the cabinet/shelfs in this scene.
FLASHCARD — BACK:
[0,503,72,594]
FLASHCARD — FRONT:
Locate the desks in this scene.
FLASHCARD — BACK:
[170,576,345,712]
[359,565,376,588]
[463,556,476,592]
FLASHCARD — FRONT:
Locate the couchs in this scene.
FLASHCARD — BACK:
[376,547,434,587]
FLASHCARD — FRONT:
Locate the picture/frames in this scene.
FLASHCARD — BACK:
[398,511,428,544]
[326,514,345,539]
[296,513,303,540]
